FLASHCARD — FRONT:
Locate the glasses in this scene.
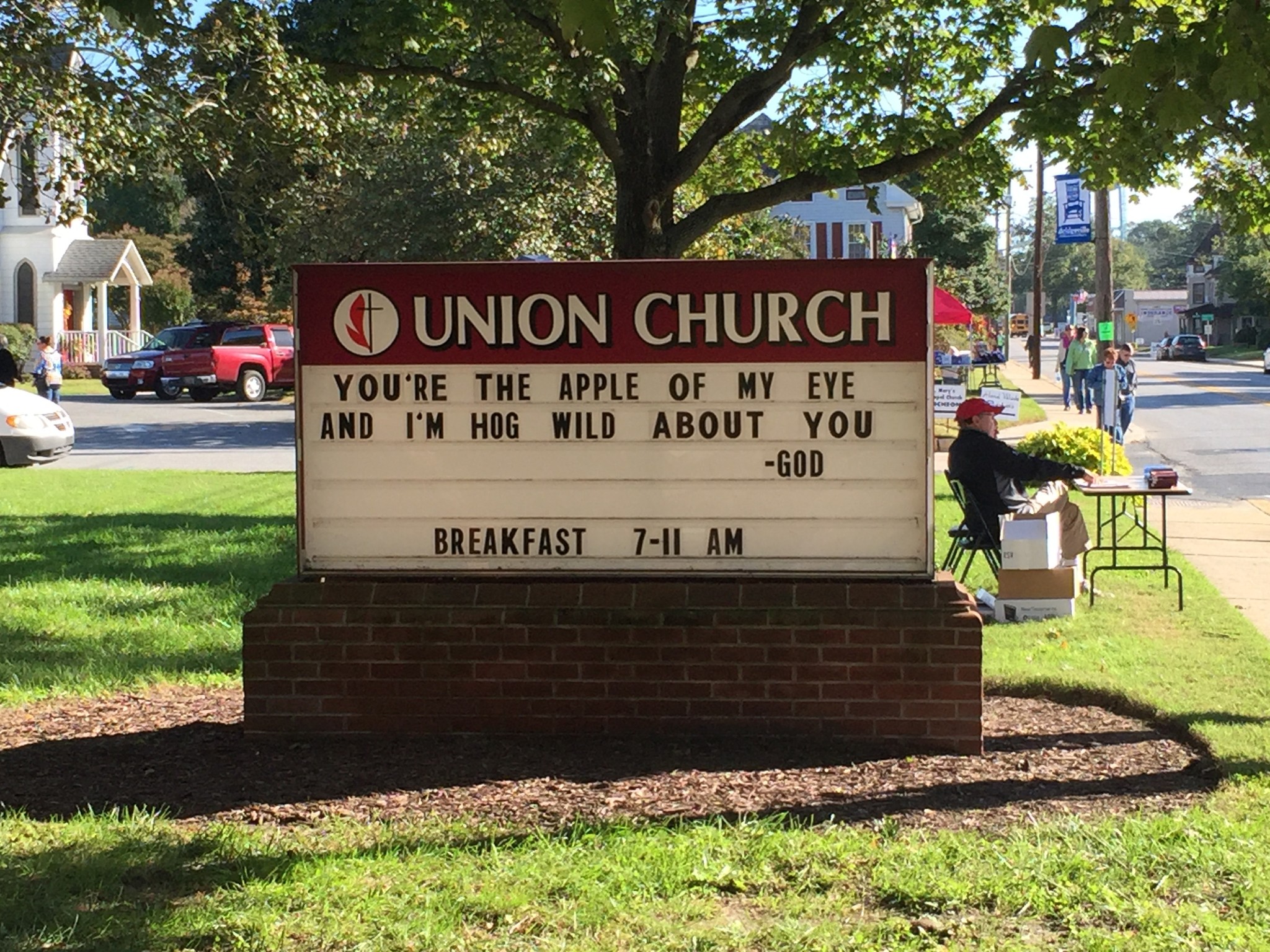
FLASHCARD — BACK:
[1105,360,1116,365]
[977,414,997,419]
[37,342,42,345]
[1122,352,1132,356]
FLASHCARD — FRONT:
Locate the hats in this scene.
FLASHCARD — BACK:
[956,398,1005,421]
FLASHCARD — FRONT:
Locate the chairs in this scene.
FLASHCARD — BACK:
[941,468,1004,591]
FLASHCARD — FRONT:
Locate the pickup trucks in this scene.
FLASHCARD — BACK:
[162,323,296,402]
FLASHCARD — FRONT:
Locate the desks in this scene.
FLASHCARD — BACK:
[1071,471,1193,612]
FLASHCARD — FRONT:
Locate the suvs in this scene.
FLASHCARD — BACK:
[102,321,257,399]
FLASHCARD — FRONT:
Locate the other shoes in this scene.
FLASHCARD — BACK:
[1086,409,1091,414]
[1078,409,1083,414]
[1064,406,1070,411]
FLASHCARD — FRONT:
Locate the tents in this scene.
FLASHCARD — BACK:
[933,284,976,398]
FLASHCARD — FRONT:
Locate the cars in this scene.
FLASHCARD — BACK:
[1169,334,1206,361]
[0,381,76,467]
[1154,337,1174,359]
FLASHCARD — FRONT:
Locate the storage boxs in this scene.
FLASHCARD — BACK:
[994,512,1081,622]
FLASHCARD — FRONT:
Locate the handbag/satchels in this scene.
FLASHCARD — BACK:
[45,370,62,389]
[1049,369,1062,385]
[1071,363,1075,376]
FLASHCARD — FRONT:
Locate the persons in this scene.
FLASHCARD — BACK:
[1065,327,1098,414]
[944,397,1102,606]
[1054,324,1082,411]
[0,333,26,388]
[1115,343,1139,442]
[1086,347,1128,446]
[964,325,1008,360]
[1022,333,1035,368]
[31,335,62,405]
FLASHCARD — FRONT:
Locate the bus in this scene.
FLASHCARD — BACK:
[1002,313,1029,337]
